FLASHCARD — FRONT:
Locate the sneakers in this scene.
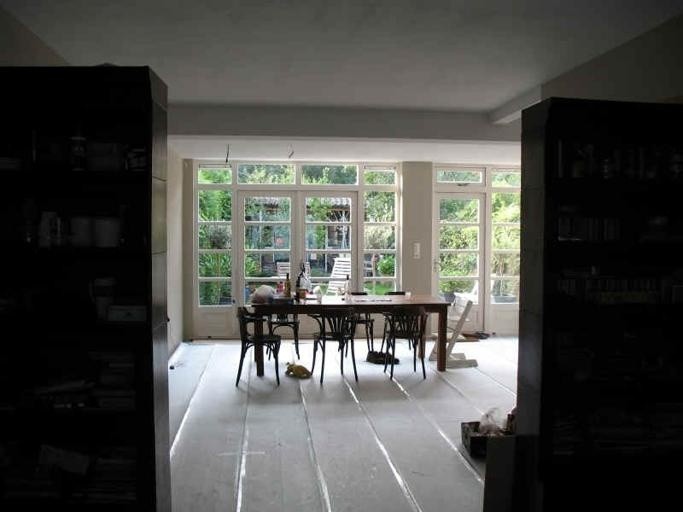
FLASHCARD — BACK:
[366,351,399,364]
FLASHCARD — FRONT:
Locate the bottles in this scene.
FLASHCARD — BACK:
[284,273,306,300]
[344,274,352,302]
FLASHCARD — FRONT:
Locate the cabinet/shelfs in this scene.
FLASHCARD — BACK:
[0,66,157,510]
[519,97,682,511]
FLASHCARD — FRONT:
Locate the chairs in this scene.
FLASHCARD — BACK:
[338,292,412,353]
[236,306,429,386]
[277,258,351,295]
[431,297,479,369]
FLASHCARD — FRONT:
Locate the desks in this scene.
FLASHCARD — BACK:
[252,294,450,372]
